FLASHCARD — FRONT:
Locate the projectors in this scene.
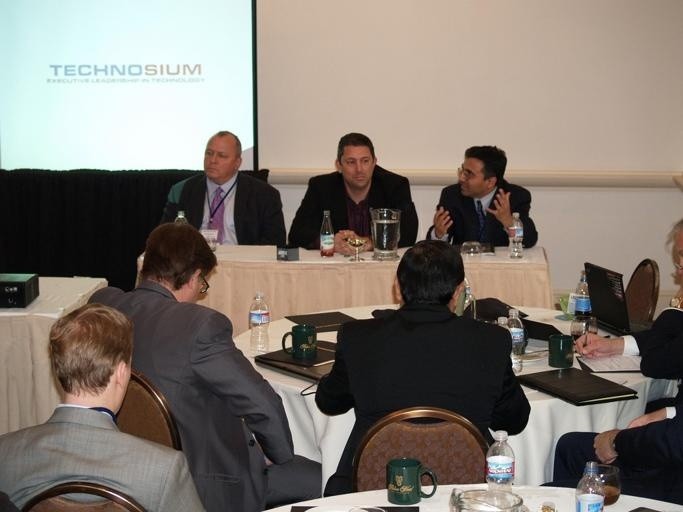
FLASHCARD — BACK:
[0,273,40,308]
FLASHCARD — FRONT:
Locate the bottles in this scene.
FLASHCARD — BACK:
[575,275,593,321]
[174,210,189,229]
[496,316,510,340]
[509,310,525,363]
[509,212,525,260]
[576,461,604,506]
[486,430,515,508]
[320,209,334,262]
[248,293,270,357]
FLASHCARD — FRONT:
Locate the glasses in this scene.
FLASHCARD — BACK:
[199,273,208,293]
[458,167,476,181]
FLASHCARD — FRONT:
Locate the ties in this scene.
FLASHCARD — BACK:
[477,199,485,228]
[208,187,224,245]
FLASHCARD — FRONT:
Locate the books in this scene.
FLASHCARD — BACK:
[514,367,638,406]
[255,340,337,383]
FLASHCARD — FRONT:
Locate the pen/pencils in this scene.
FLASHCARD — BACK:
[585,322,589,347]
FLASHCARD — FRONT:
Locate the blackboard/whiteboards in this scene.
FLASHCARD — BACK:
[252,0,683,188]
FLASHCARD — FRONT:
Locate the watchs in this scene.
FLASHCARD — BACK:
[669,296,683,309]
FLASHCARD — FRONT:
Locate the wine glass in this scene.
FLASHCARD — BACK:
[344,237,367,265]
[570,316,599,362]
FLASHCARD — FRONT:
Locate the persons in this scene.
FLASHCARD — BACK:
[315,237,531,499]
[287,133,419,257]
[86,223,322,511]
[552,401,682,503]
[574,219,683,414]
[426,145,538,249]
[156,131,286,246]
[0,303,207,512]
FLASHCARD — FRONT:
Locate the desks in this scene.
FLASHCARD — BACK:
[233,303,683,498]
[0,277,112,436]
[135,245,556,339]
[260,483,683,512]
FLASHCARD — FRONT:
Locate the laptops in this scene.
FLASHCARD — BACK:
[584,262,654,336]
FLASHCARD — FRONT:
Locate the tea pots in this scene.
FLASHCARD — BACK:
[462,285,477,319]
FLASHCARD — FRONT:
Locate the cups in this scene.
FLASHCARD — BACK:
[369,208,401,261]
[547,336,574,369]
[387,456,436,505]
[206,238,216,255]
[454,489,523,512]
[282,323,317,361]
[462,242,483,260]
[594,464,622,504]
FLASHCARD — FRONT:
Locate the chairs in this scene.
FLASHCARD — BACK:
[22,482,148,512]
[351,406,494,493]
[114,368,182,451]
[625,259,661,321]
